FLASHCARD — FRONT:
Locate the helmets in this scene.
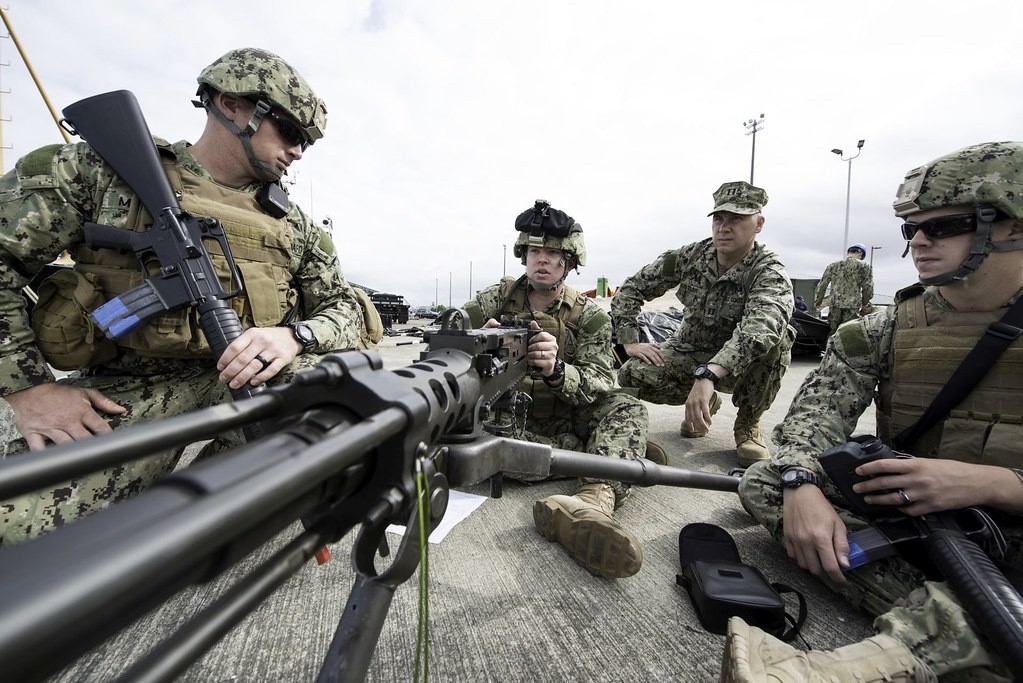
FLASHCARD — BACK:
[891,140,1023,223]
[197,47,328,146]
[513,207,587,266]
[847,242,868,261]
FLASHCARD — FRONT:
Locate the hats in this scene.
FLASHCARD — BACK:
[706,180,770,217]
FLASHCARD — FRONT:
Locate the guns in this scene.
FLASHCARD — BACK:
[816,434,1023,680]
[56,84,332,568]
[1,312,544,682]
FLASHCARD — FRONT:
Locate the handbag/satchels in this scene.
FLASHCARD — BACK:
[675,522,808,642]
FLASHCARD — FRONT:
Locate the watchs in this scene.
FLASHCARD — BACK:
[693,364,719,386]
[547,358,565,381]
[780,468,821,492]
[286,322,316,356]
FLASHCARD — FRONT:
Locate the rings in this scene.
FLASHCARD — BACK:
[541,351,545,359]
[900,492,910,504]
[256,355,267,366]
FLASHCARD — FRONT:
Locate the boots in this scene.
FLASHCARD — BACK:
[680,389,722,439]
[733,412,771,468]
[531,481,644,580]
[644,440,669,467]
[719,615,939,683]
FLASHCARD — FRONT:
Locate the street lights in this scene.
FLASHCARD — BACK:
[743,113,766,186]
[830,139,865,262]
[869,246,883,266]
[502,244,507,277]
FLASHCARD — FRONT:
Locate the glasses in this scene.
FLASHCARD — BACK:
[244,95,311,156]
[901,210,1013,242]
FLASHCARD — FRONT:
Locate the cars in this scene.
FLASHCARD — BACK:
[414,308,439,319]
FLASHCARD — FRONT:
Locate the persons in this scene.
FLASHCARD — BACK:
[610,181,797,469]
[0,47,363,548]
[452,199,668,578]
[795,296,809,313]
[814,244,875,338]
[720,138,1023,683]
